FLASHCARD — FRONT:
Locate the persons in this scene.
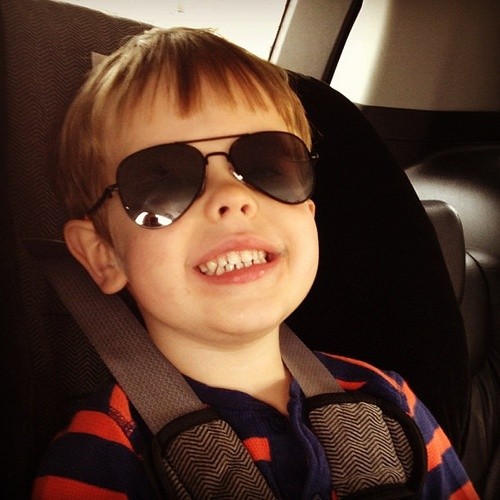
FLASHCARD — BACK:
[29,25,480,500]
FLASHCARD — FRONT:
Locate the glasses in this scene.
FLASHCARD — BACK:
[82,129,320,229]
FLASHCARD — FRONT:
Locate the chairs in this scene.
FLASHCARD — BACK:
[1,0,500,500]
